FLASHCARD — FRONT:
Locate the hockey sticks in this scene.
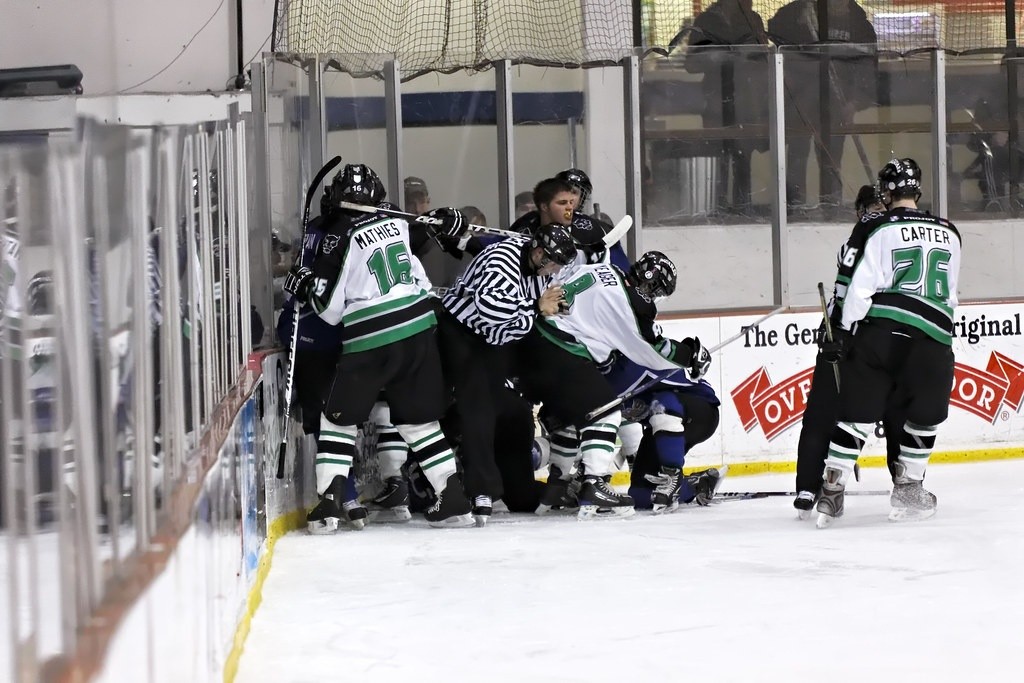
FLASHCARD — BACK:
[591,201,627,471]
[816,280,861,483]
[736,0,857,202]
[338,199,635,255]
[801,1,876,188]
[275,153,342,480]
[713,490,891,498]
[585,306,789,421]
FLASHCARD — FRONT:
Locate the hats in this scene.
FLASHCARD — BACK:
[404,176,428,197]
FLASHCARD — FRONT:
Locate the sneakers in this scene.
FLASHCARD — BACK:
[541,464,578,513]
[307,475,346,534]
[342,500,371,529]
[689,469,719,506]
[887,483,938,520]
[643,468,685,513]
[425,474,476,528]
[793,490,817,519]
[817,482,845,528]
[579,475,635,522]
[366,478,411,523]
[471,494,493,526]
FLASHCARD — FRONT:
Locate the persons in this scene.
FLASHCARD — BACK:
[686,1,769,222]
[1,166,263,541]
[767,0,877,222]
[793,159,963,510]
[966,48,1024,213]
[275,163,720,521]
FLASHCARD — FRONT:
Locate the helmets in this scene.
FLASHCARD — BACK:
[854,185,882,211]
[533,221,578,265]
[334,164,386,208]
[319,185,340,216]
[559,169,592,211]
[629,251,677,299]
[873,158,922,205]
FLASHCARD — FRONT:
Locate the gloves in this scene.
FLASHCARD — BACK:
[821,327,850,363]
[284,263,328,302]
[680,337,712,378]
[422,205,466,241]
[435,234,474,260]
